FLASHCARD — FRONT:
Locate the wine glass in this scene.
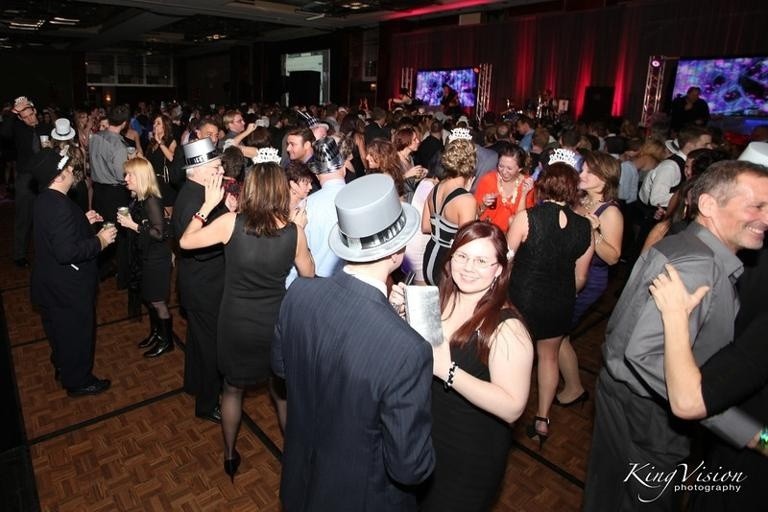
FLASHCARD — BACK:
[485,191,497,209]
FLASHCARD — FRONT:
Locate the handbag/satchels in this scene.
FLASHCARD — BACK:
[405,284,444,348]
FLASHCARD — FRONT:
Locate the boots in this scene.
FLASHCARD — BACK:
[138,313,174,358]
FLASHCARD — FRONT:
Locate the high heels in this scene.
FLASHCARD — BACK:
[224,452,240,485]
[552,389,590,410]
[526,416,550,451]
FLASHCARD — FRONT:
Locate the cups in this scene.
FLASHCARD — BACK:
[117,206,129,217]
[102,222,115,230]
[126,146,135,154]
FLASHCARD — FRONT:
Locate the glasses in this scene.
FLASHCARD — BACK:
[452,251,499,270]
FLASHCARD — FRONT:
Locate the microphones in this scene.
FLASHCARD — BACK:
[685,97,696,111]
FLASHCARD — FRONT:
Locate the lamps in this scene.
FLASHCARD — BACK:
[650,56,662,67]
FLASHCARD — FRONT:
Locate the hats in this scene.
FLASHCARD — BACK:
[28,150,73,192]
[329,173,421,264]
[665,138,679,155]
[51,118,76,141]
[181,137,225,169]
[307,137,347,174]
[737,141,768,168]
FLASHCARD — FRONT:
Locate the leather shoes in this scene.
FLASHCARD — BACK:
[67,379,111,397]
[207,406,222,423]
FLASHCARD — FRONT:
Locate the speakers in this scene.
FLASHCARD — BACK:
[582,87,614,121]
[289,71,320,106]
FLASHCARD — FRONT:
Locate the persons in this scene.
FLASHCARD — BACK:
[0,82,767,511]
